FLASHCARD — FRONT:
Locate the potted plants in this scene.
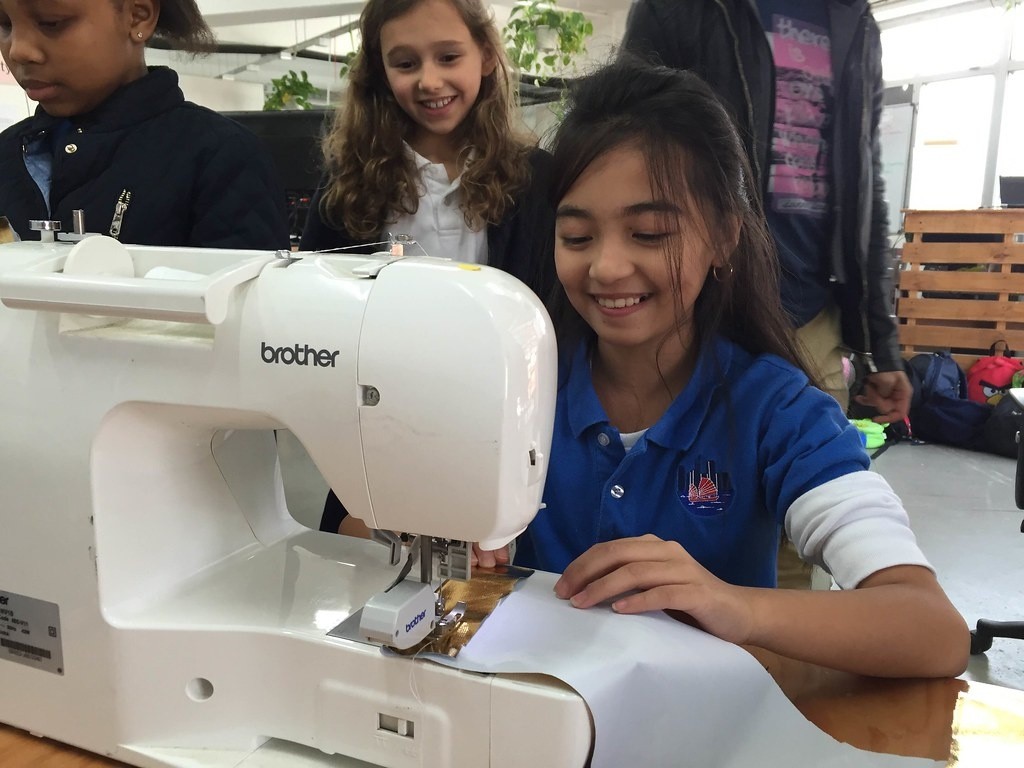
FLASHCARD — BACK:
[500,0,594,123]
[263,69,321,111]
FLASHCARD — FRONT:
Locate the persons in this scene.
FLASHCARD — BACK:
[298,0,554,279]
[615,0,913,423]
[338,64,971,679]
[0,1,290,250]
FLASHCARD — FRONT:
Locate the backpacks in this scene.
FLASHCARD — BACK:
[966,337,1024,407]
[906,347,969,403]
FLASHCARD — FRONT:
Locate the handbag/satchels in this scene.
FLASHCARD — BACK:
[908,392,1024,460]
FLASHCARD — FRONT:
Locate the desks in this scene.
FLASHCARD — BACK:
[0,564,1024,768]
